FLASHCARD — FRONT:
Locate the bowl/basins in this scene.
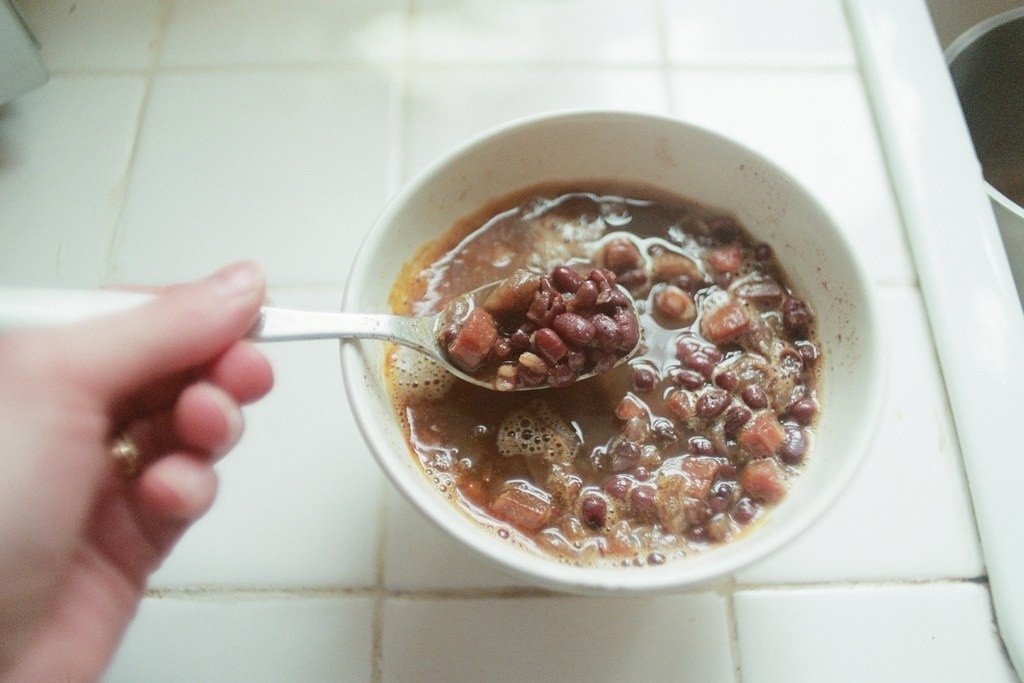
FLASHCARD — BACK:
[339,107,886,600]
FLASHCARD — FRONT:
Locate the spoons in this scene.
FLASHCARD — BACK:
[1,268,641,391]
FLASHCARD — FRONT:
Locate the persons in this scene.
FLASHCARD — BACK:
[0,261,274,683]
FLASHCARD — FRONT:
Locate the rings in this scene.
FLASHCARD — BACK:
[106,425,144,481]
